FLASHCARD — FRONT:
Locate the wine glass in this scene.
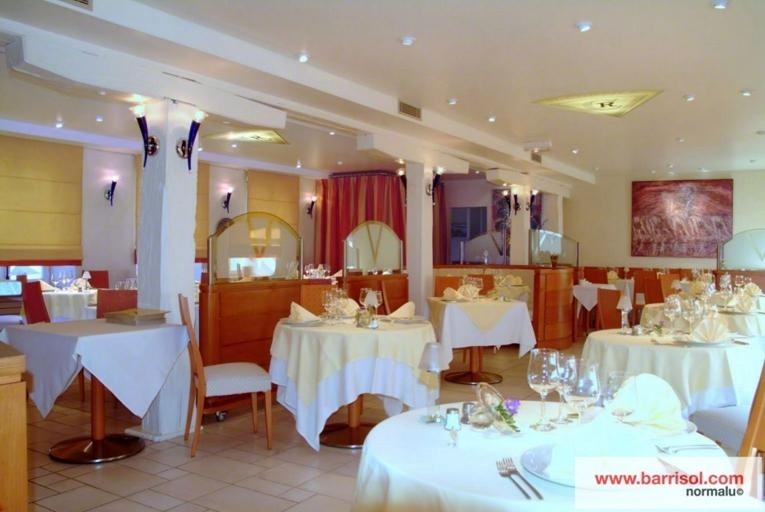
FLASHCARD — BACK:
[526,347,560,432]
[718,273,732,303]
[50,270,73,292]
[304,264,330,279]
[682,299,700,337]
[734,275,744,293]
[552,353,578,424]
[562,359,599,430]
[359,289,382,328]
[664,295,681,335]
[321,285,348,327]
[604,370,636,425]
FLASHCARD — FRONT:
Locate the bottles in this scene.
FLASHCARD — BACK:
[115,276,137,290]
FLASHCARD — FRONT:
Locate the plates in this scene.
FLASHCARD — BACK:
[281,319,321,325]
[521,443,631,492]
[687,335,729,346]
[385,315,425,324]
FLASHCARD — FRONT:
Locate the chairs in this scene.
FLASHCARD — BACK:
[571,259,761,330]
[732,358,765,498]
[177,293,273,459]
[1,262,137,324]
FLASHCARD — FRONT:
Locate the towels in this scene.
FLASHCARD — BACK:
[616,374,682,428]
[685,317,734,344]
[443,287,460,298]
[389,299,416,318]
[459,284,481,300]
[289,302,317,319]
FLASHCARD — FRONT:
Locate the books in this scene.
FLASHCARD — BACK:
[103,308,171,326]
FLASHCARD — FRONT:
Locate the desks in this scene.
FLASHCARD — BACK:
[0,340,30,512]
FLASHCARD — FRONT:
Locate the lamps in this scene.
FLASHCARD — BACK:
[297,52,309,63]
[447,97,459,105]
[501,186,538,216]
[395,165,445,188]
[575,20,592,33]
[105,101,316,217]
[400,36,416,47]
[710,0,728,9]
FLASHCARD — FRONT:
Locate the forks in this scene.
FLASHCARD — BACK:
[503,457,544,500]
[496,460,531,500]
